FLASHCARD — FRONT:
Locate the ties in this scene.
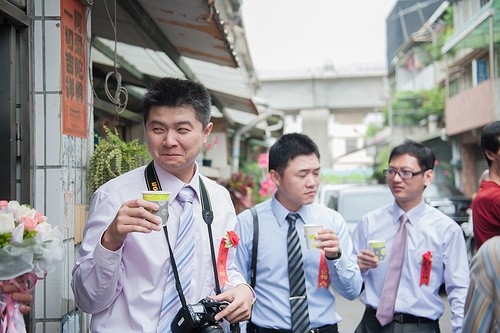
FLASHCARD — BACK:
[157,188,196,333]
[376,215,409,326]
[285,214,311,333]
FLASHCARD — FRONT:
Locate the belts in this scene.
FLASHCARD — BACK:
[365,308,434,324]
[246,321,337,333]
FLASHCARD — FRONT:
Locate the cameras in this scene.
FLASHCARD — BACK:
[171,298,229,333]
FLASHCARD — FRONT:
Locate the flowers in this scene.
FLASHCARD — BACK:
[419,251,432,266]
[0,200,65,333]
[221,230,240,248]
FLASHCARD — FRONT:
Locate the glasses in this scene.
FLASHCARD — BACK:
[383,167,429,179]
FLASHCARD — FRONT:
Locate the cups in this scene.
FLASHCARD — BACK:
[141,191,172,227]
[303,224,324,250]
[368,239,386,261]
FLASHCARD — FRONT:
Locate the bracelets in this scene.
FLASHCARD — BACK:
[325,248,342,261]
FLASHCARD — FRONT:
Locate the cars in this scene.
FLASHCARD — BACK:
[315,183,475,293]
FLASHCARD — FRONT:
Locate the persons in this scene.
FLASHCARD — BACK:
[72,78,256,333]
[226,134,363,333]
[352,138,470,333]
[462,120,500,333]
[0,280,33,321]
